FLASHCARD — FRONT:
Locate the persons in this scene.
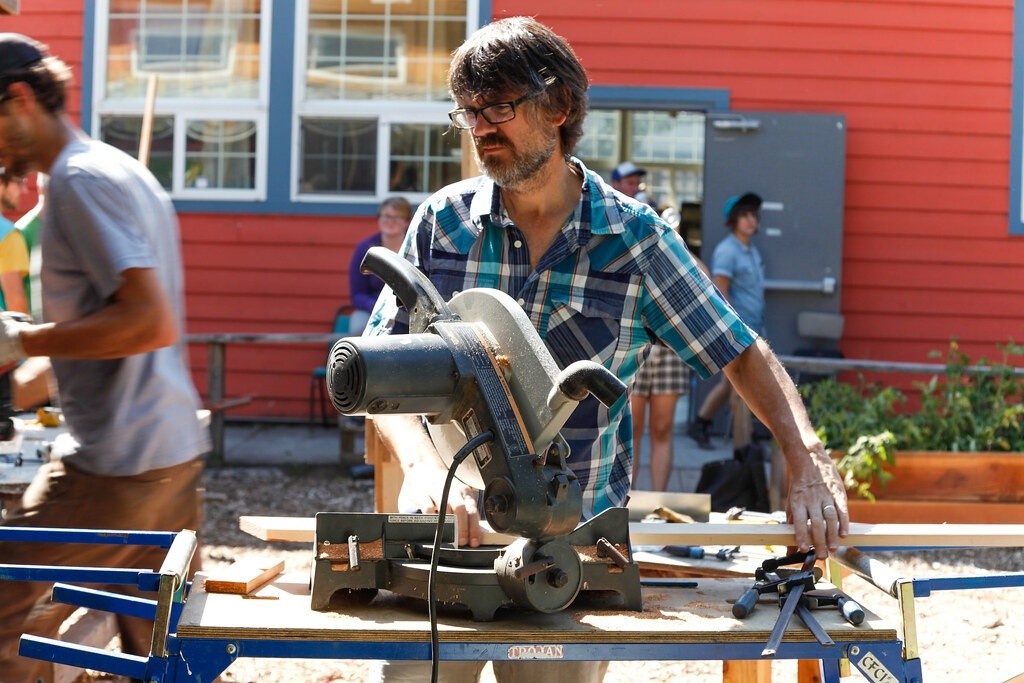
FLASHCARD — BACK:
[349,196,412,340]
[14,171,49,325]
[0,33,212,683]
[687,192,768,461]
[633,207,711,491]
[361,15,850,683]
[612,161,649,198]
[0,163,54,416]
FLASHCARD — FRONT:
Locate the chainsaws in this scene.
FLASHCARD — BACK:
[307,243,643,624]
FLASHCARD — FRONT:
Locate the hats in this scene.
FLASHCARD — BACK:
[723,192,763,220]
[0,33,42,78]
[611,161,647,180]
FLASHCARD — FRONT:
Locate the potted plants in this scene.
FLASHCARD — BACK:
[781,336,1024,525]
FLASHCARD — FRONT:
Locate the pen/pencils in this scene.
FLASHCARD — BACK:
[641,582,698,588]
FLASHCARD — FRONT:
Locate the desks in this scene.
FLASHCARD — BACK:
[632,512,828,683]
[0,406,213,683]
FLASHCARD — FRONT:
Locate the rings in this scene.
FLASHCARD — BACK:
[822,505,836,514]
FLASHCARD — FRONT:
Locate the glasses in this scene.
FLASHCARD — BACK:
[448,95,528,129]
[9,174,27,187]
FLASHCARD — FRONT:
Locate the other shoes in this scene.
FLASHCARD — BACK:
[350,463,374,480]
[687,420,716,449]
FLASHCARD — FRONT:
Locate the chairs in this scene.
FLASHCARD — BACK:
[307,306,354,435]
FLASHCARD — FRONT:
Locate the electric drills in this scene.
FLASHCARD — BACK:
[664,545,717,558]
